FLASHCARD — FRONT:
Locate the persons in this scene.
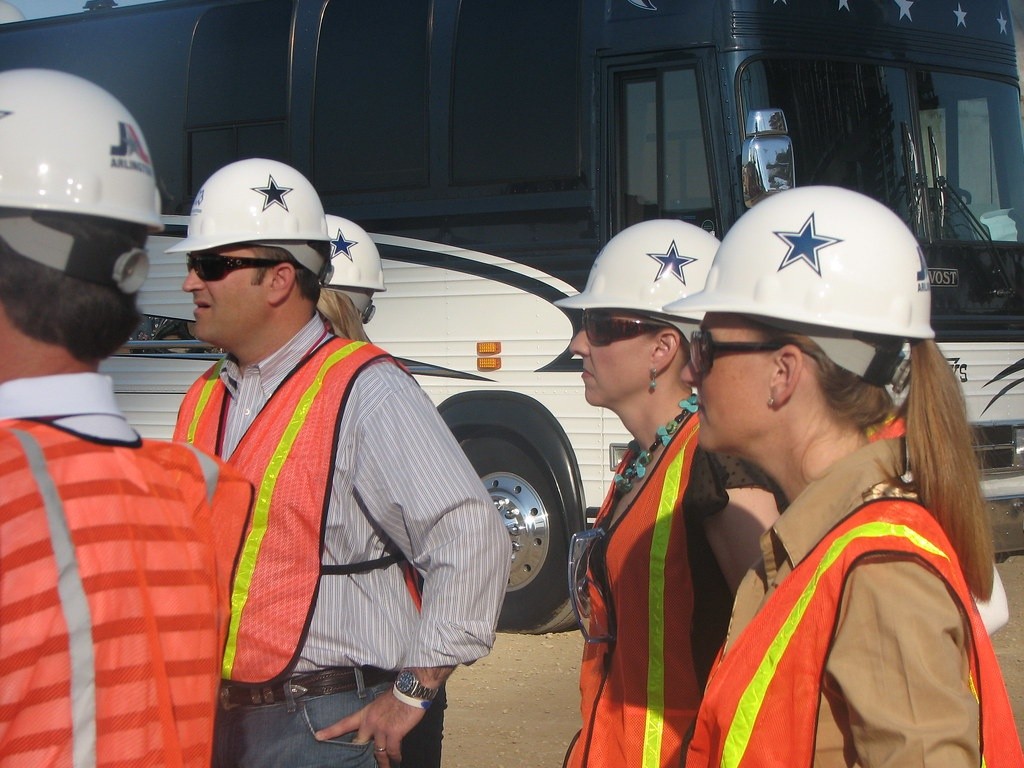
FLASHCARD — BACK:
[549,217,781,768]
[659,186,1024,768]
[0,68,511,768]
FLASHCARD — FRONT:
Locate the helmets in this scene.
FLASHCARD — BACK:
[164,158,330,254]
[319,214,387,291]
[0,69,164,234]
[663,185,935,339]
[553,219,723,320]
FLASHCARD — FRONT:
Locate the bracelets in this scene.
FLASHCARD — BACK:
[392,686,431,709]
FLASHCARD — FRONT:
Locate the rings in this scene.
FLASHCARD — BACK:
[374,747,386,752]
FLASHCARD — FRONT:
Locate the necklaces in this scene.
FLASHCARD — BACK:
[615,392,698,492]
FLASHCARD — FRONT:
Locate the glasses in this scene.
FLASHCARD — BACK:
[690,331,819,381]
[581,309,688,347]
[567,528,617,643]
[185,253,303,281]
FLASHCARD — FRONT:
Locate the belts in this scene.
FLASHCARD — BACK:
[217,665,397,711]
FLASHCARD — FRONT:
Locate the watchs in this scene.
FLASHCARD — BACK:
[395,670,439,700]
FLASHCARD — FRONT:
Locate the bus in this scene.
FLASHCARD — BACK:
[1,0,1024,644]
